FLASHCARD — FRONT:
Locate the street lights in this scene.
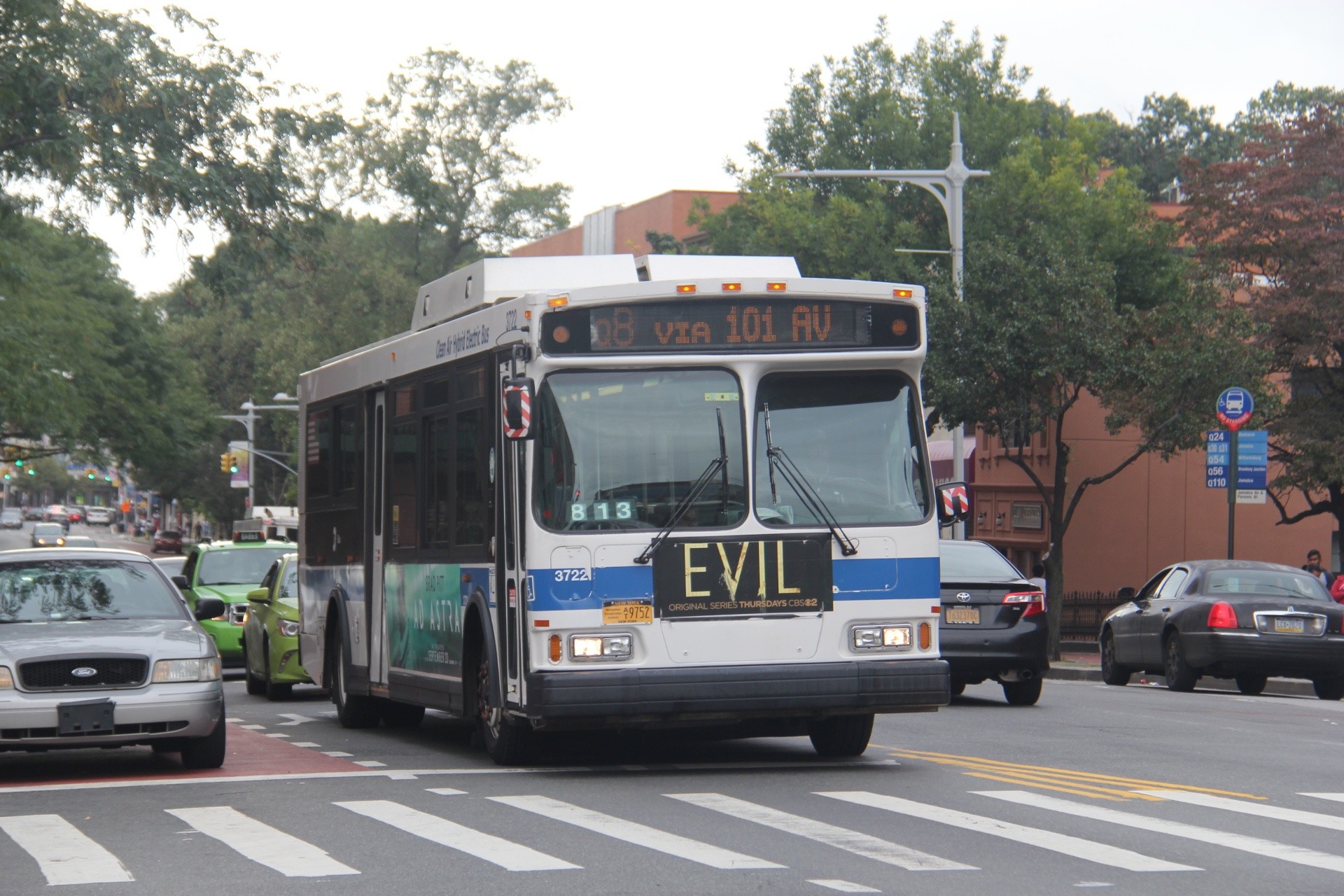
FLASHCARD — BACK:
[775,113,990,545]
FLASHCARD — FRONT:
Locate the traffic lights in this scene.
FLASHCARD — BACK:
[27,466,34,476]
[227,455,239,474]
[84,468,96,481]
[14,460,23,467]
[4,468,12,480]
[220,453,230,471]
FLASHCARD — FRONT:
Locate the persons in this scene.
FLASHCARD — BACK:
[1331,574,1344,634]
[1301,549,1329,590]
[1306,564,1320,578]
[194,522,203,542]
[1028,565,1046,597]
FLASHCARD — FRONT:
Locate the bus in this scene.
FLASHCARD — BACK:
[299,253,972,770]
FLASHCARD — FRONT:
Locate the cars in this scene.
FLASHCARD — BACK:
[0,549,232,776]
[937,535,1051,706]
[0,501,321,702]
[1097,558,1344,702]
